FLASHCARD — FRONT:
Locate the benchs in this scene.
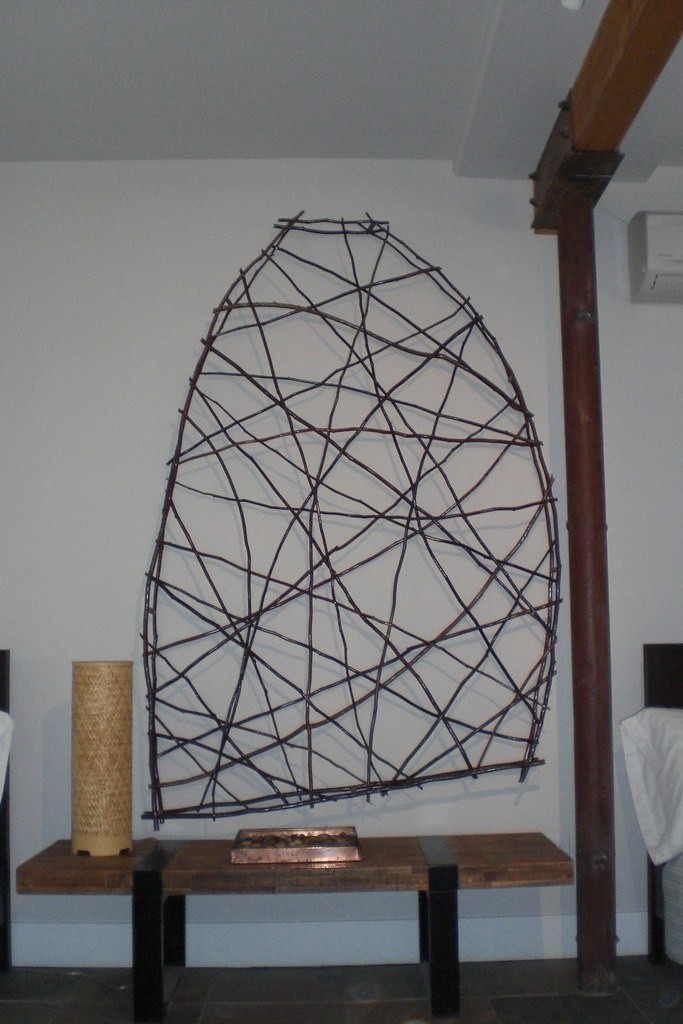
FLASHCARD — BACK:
[17,833,572,1024]
